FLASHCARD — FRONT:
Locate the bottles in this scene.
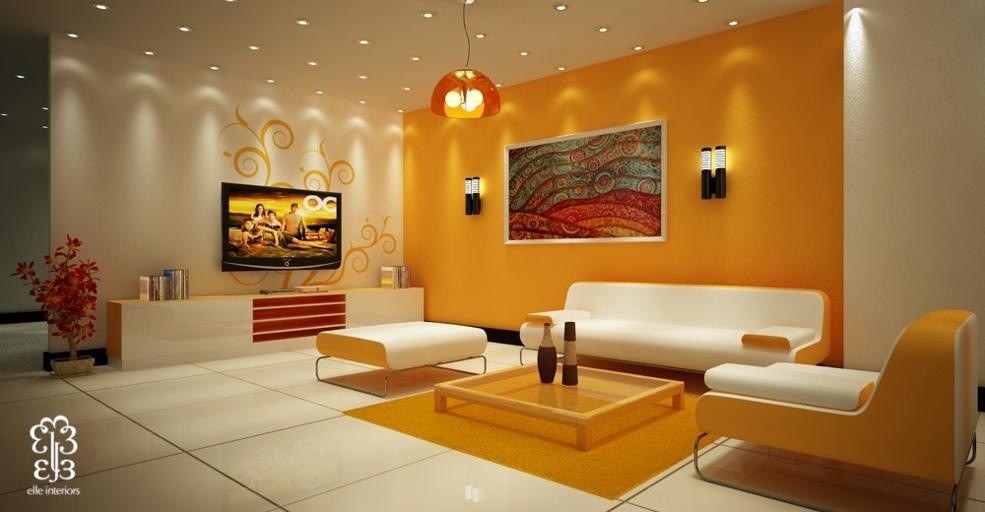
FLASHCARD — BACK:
[537,323,557,383]
[562,322,578,386]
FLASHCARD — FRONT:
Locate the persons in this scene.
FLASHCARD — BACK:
[241,202,338,252]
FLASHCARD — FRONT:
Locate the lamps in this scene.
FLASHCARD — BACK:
[701,146,726,199]
[465,177,481,215]
[431,0,500,119]
[504,119,668,244]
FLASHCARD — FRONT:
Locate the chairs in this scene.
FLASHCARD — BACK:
[694,309,978,512]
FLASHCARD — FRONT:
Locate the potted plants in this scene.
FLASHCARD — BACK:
[9,234,101,375]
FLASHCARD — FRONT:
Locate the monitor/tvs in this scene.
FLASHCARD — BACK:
[220,181,342,270]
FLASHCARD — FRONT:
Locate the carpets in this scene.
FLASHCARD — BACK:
[343,355,721,500]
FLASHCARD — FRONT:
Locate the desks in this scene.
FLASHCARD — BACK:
[107,287,425,370]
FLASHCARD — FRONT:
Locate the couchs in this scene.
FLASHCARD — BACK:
[520,281,831,374]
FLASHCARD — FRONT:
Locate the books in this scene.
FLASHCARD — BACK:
[138,268,190,302]
[380,265,412,289]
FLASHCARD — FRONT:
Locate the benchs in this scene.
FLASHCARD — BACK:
[316,321,487,398]
